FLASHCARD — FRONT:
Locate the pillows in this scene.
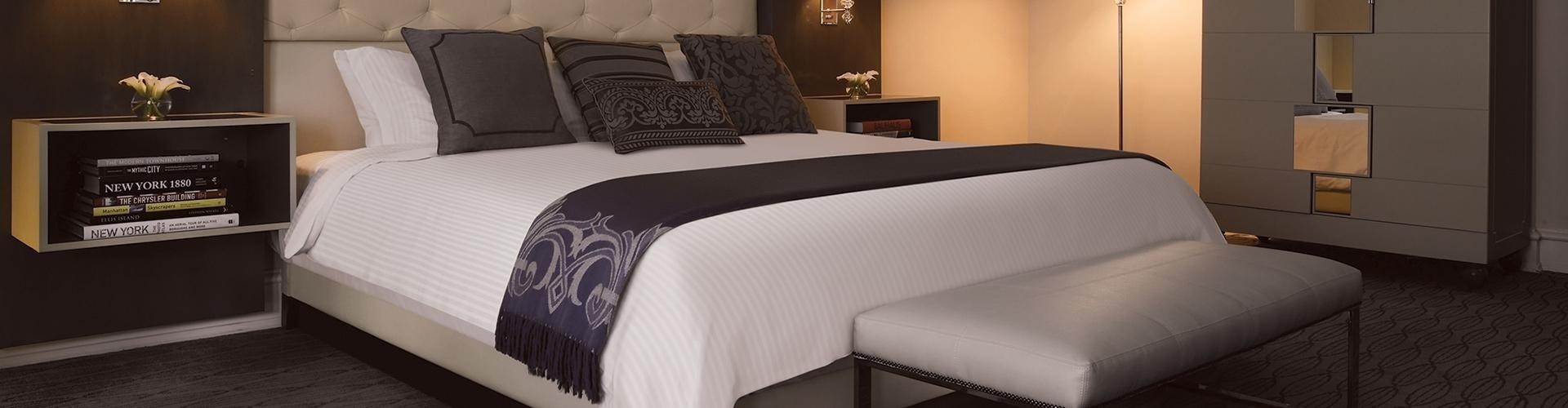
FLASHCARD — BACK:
[583,78,746,155]
[543,40,694,140]
[401,25,579,154]
[673,32,818,136]
[547,35,676,142]
[333,47,439,146]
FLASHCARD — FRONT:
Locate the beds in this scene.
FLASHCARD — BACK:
[262,40,1213,405]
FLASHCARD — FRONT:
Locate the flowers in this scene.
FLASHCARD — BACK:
[836,70,879,94]
[117,71,191,120]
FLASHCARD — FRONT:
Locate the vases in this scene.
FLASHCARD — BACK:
[132,92,174,121]
[849,86,867,97]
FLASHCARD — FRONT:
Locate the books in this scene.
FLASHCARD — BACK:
[847,119,914,138]
[62,152,240,241]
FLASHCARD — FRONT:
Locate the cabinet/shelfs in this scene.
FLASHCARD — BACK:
[804,95,943,143]
[10,110,299,257]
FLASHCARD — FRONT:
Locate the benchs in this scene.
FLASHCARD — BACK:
[852,239,1366,408]
